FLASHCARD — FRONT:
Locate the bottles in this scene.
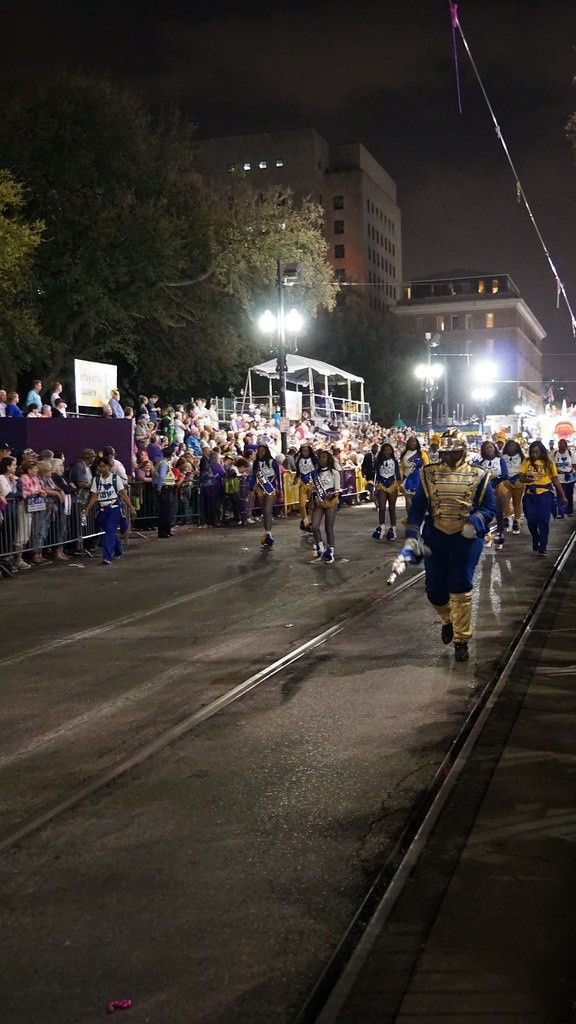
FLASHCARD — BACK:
[81,509,87,526]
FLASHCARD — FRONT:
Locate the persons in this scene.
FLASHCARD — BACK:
[102,390,125,418]
[125,395,483,538]
[519,441,568,556]
[103,447,128,485]
[372,444,403,540]
[80,458,136,565]
[406,427,497,662]
[549,440,576,518]
[478,441,511,550]
[311,451,340,563]
[0,443,99,573]
[0,390,23,417]
[249,444,281,547]
[26,381,66,417]
[485,431,529,458]
[501,440,526,534]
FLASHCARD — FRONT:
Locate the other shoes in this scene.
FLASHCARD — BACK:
[144,514,263,538]
[372,524,386,539]
[310,555,322,563]
[326,557,335,564]
[507,522,513,533]
[453,642,469,660]
[486,540,493,548]
[260,534,274,548]
[441,622,454,644]
[9,548,69,570]
[114,553,120,559]
[533,542,547,556]
[497,543,503,550]
[386,525,398,541]
[104,558,113,564]
[513,529,520,535]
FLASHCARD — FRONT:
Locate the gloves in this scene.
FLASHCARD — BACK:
[462,524,477,539]
[406,539,421,556]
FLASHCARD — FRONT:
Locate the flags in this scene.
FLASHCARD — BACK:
[540,386,554,403]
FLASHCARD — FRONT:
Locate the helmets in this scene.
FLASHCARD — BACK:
[438,428,467,460]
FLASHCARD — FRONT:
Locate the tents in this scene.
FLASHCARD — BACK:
[248,354,365,418]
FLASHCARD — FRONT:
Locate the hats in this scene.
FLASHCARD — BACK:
[82,448,97,458]
[162,448,174,456]
[0,442,15,451]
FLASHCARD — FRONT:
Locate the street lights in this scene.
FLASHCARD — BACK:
[256,258,303,454]
[415,331,443,424]
